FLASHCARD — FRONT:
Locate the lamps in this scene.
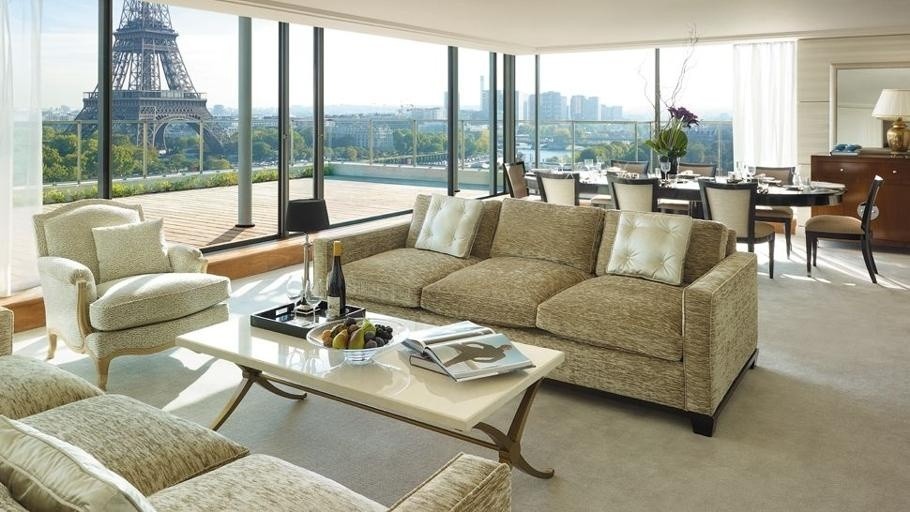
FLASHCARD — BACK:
[282,198,330,299]
[872,87,909,155]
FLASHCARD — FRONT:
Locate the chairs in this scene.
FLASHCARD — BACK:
[29,198,233,393]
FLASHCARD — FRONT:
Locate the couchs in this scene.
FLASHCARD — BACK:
[1,305,514,510]
[312,193,760,439]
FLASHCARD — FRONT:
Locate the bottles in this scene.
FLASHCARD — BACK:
[324,241,347,319]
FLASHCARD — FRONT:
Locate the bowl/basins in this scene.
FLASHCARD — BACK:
[304,318,409,367]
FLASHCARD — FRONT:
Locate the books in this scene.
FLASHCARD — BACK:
[398,320,536,383]
[409,351,449,376]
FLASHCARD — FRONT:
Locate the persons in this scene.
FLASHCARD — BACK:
[442,340,513,368]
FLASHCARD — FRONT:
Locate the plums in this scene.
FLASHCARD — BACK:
[383,339,388,344]
[344,318,356,328]
[364,331,375,341]
[373,337,385,348]
[376,324,393,339]
[365,341,377,349]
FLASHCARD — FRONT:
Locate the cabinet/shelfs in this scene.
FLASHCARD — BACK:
[811,151,909,254]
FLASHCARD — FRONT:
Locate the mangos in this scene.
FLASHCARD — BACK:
[332,329,348,349]
[348,329,364,350]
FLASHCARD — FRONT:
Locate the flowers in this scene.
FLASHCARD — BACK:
[644,99,700,160]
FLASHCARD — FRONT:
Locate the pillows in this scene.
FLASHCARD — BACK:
[89,217,174,283]
[2,415,147,510]
[412,193,485,260]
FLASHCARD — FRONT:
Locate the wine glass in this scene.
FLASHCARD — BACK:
[284,275,304,326]
[303,278,323,328]
[728,159,812,194]
[558,155,671,185]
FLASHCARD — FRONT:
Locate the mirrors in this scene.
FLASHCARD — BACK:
[828,62,910,153]
